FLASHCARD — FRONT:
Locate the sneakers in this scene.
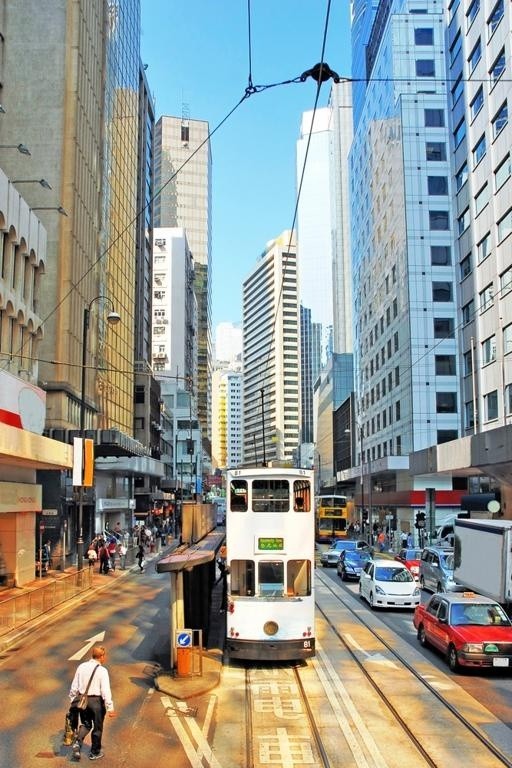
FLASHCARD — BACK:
[72,741,80,760]
[89,752,103,760]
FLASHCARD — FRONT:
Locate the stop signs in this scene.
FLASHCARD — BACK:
[38,521,46,533]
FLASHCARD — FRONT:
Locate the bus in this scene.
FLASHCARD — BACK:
[223,466,318,665]
[314,494,354,543]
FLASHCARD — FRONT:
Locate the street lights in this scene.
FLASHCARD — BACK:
[343,419,364,534]
[75,295,121,549]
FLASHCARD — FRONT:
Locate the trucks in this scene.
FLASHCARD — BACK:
[453,516,512,606]
[429,516,453,547]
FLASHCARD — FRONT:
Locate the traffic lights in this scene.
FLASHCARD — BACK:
[414,514,417,528]
[419,513,425,528]
[155,501,161,516]
[168,503,174,516]
[363,510,368,520]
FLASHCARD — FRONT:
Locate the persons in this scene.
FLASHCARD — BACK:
[86,512,181,574]
[70,646,116,761]
[219,542,227,565]
[344,518,429,552]
[231,495,305,512]
[44,540,51,571]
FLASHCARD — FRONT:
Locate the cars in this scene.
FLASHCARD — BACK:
[338,548,374,579]
[358,559,421,611]
[414,591,509,672]
[393,547,424,581]
[320,538,370,568]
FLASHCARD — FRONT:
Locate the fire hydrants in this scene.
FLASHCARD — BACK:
[167,534,173,545]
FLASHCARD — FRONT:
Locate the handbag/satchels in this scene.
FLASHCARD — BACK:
[70,695,88,711]
[63,713,78,746]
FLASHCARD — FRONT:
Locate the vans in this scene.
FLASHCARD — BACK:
[418,545,465,594]
